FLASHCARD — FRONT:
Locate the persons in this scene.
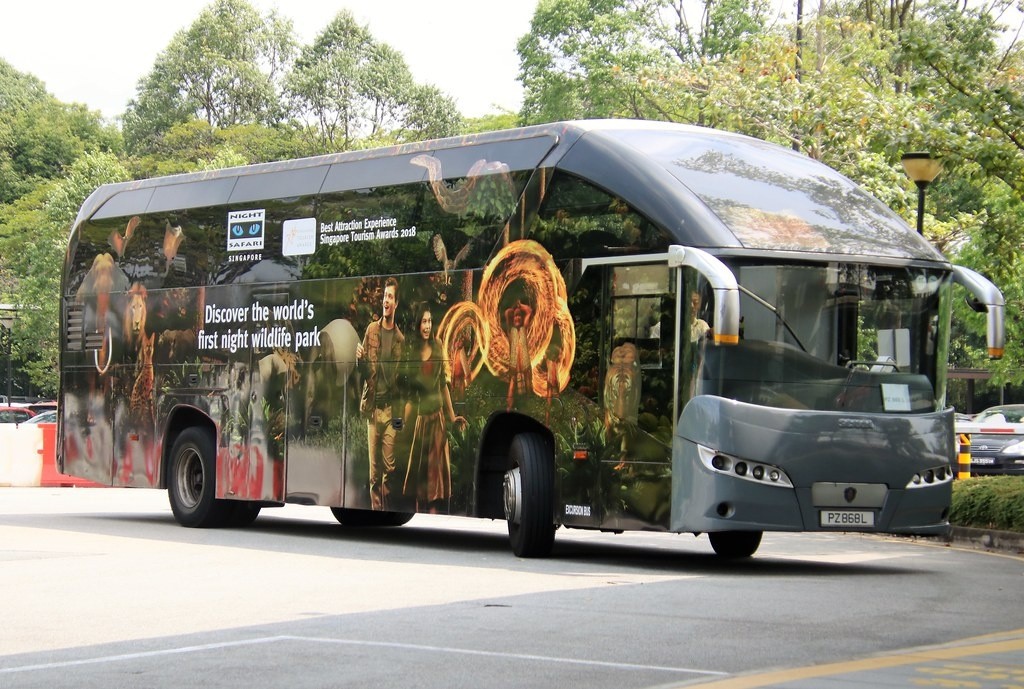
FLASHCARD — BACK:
[651,290,710,341]
[355,277,408,512]
[396,303,469,514]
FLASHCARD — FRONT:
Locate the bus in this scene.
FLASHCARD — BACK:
[54,119,1011,573]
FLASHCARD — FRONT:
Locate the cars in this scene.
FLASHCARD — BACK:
[0,392,59,423]
[954,405,1024,479]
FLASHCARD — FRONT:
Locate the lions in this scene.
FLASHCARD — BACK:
[122,279,147,357]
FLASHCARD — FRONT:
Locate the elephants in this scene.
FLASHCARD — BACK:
[73,254,128,425]
[211,318,366,459]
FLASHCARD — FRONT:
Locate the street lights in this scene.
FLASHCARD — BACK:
[900,149,947,240]
